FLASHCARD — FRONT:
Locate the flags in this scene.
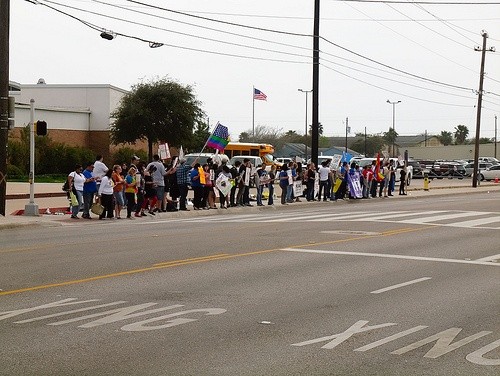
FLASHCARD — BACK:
[254,88,268,102]
[206,123,231,153]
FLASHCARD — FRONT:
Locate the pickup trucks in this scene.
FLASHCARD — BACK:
[274,156,414,187]
[226,155,272,181]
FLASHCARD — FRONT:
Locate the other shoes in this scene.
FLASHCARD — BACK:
[141,212,147,216]
[127,216,135,219]
[153,208,158,212]
[308,192,407,202]
[99,217,105,219]
[117,217,121,219]
[82,213,92,219]
[189,198,302,210]
[110,217,117,220]
[148,210,155,215]
[162,207,189,212]
[135,213,141,217]
[158,209,162,212]
[71,215,79,219]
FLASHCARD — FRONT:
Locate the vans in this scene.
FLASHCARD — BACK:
[183,153,230,166]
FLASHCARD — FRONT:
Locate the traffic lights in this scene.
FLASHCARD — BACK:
[35,120,47,136]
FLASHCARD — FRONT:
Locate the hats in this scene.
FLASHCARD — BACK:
[222,157,227,162]
[132,156,140,161]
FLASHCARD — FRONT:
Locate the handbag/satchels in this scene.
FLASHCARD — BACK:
[91,198,104,215]
[152,181,158,189]
[62,173,75,191]
[69,191,79,207]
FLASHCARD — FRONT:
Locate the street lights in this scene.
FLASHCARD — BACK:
[386,99,401,157]
[298,88,314,164]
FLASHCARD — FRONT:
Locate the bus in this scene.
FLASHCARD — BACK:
[214,142,275,165]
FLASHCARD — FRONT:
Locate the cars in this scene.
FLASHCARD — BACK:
[410,157,500,182]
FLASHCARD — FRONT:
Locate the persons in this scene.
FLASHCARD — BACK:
[67,154,407,219]
